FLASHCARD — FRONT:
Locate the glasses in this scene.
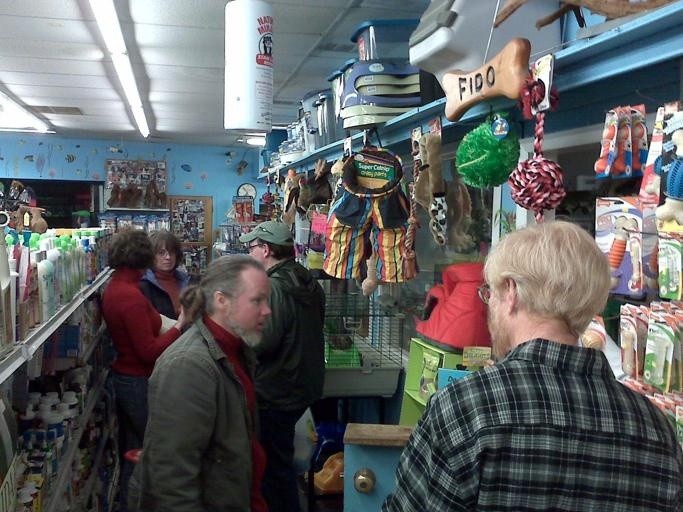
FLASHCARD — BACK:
[476,282,497,304]
[246,245,260,255]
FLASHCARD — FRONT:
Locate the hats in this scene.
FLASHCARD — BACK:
[238,220,297,244]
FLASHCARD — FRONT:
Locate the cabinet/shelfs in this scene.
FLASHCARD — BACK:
[398,336,482,426]
[0,266,122,511]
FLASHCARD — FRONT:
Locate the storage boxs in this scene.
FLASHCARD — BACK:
[308,18,418,151]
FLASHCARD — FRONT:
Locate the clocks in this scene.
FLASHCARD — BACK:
[235,183,257,201]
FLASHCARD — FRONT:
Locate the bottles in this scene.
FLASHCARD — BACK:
[8,227,113,349]
[18,381,118,512]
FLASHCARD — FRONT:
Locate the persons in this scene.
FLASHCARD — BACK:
[126,254,274,511]
[139,229,200,320]
[238,220,326,512]
[380,219,683,512]
[102,228,186,512]
